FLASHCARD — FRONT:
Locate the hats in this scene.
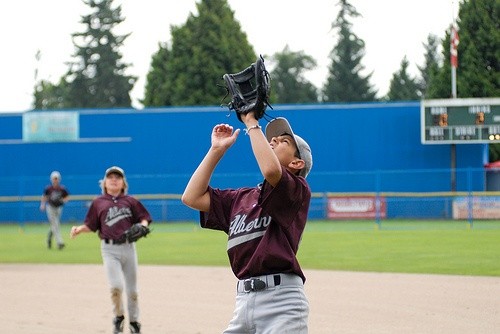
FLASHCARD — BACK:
[265,117,313,178]
[106,166,124,177]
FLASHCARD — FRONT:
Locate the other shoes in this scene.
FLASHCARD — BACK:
[113,316,124,334]
[48,240,51,249]
[129,321,140,334]
[57,244,65,250]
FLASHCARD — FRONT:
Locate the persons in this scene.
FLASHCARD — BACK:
[70,167,153,334]
[182,54,311,334]
[41,171,70,250]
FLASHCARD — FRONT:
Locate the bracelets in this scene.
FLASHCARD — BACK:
[243,125,261,135]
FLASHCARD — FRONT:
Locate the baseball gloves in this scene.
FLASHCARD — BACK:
[123,224,152,243]
[222,57,269,124]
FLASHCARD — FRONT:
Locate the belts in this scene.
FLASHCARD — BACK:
[106,238,126,244]
[243,275,280,292]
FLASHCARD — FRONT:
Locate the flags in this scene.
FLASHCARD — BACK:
[450,26,459,68]
